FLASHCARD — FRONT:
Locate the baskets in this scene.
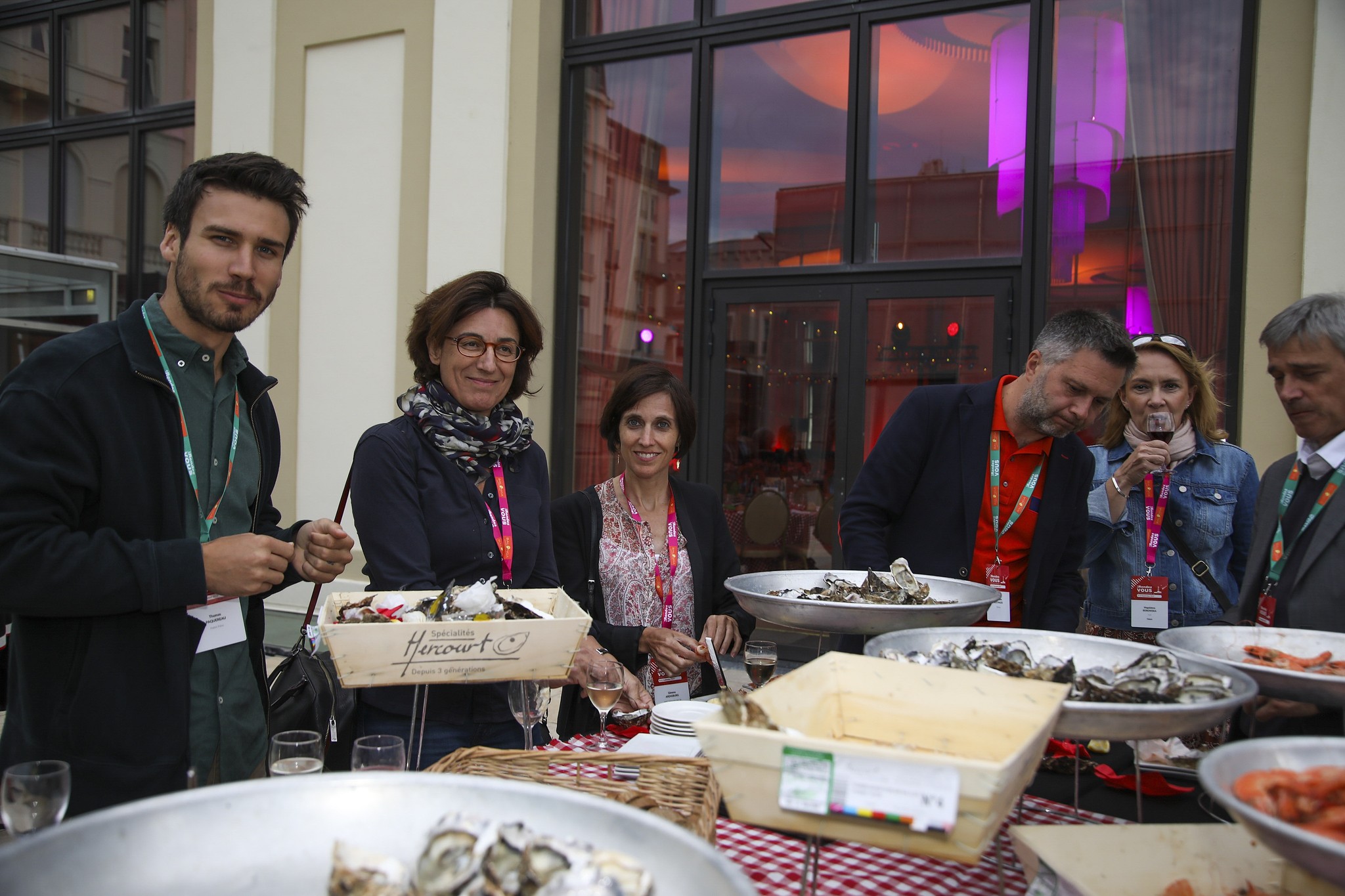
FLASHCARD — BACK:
[417,746,720,850]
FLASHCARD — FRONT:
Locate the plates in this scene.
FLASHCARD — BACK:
[1159,620,1345,710]
[721,569,1003,636]
[648,700,720,738]
[0,771,755,896]
[1198,731,1345,883]
[864,627,1255,744]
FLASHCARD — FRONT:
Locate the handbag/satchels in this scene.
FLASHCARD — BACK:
[266,633,355,771]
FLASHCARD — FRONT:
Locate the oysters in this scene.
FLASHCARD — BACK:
[765,557,958,605]
[322,816,653,896]
[879,636,1232,702]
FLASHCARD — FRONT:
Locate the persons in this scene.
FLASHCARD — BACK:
[838,309,1139,658]
[548,365,758,746]
[1203,291,1345,746]
[0,149,354,818]
[1075,333,1263,752]
[351,270,659,773]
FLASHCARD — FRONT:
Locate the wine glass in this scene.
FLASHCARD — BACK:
[506,671,551,749]
[744,638,777,689]
[2,759,69,838]
[1147,410,1181,477]
[351,734,408,769]
[267,730,325,777]
[586,659,624,752]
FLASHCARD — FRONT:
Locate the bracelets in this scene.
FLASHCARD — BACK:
[595,648,610,655]
[1111,473,1130,499]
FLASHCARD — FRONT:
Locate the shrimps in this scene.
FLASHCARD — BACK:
[1235,764,1345,844]
[1242,644,1345,676]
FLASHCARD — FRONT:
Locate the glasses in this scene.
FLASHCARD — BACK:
[1130,333,1193,357]
[442,335,527,363]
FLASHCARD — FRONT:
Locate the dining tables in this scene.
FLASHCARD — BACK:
[529,676,1345,896]
